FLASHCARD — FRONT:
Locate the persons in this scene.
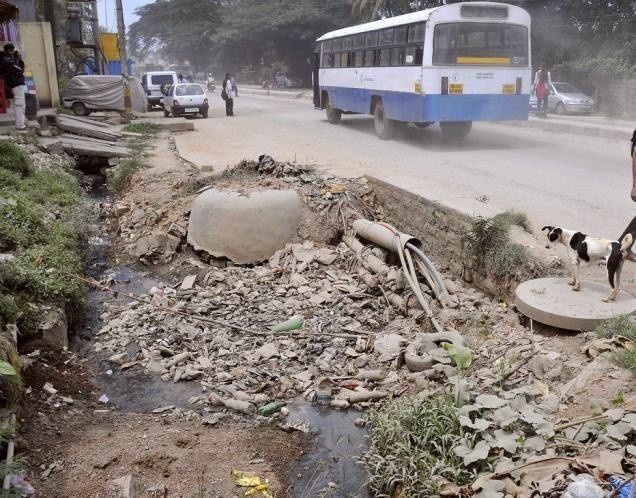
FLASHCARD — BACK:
[271,64,278,88]
[0,43,26,130]
[532,63,552,119]
[206,72,215,83]
[178,74,186,83]
[222,73,240,116]
[630,127,636,203]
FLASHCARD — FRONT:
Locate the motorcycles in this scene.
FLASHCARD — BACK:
[206,81,217,92]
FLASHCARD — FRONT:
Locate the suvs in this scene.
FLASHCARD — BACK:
[140,71,178,112]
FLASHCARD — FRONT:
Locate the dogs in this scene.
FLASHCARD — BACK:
[541,225,633,302]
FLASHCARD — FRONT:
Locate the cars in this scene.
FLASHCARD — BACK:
[275,71,303,89]
[162,83,209,118]
[529,82,594,115]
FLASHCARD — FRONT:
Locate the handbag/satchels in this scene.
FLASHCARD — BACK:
[221,90,228,100]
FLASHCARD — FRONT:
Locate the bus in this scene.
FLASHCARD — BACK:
[307,1,533,140]
